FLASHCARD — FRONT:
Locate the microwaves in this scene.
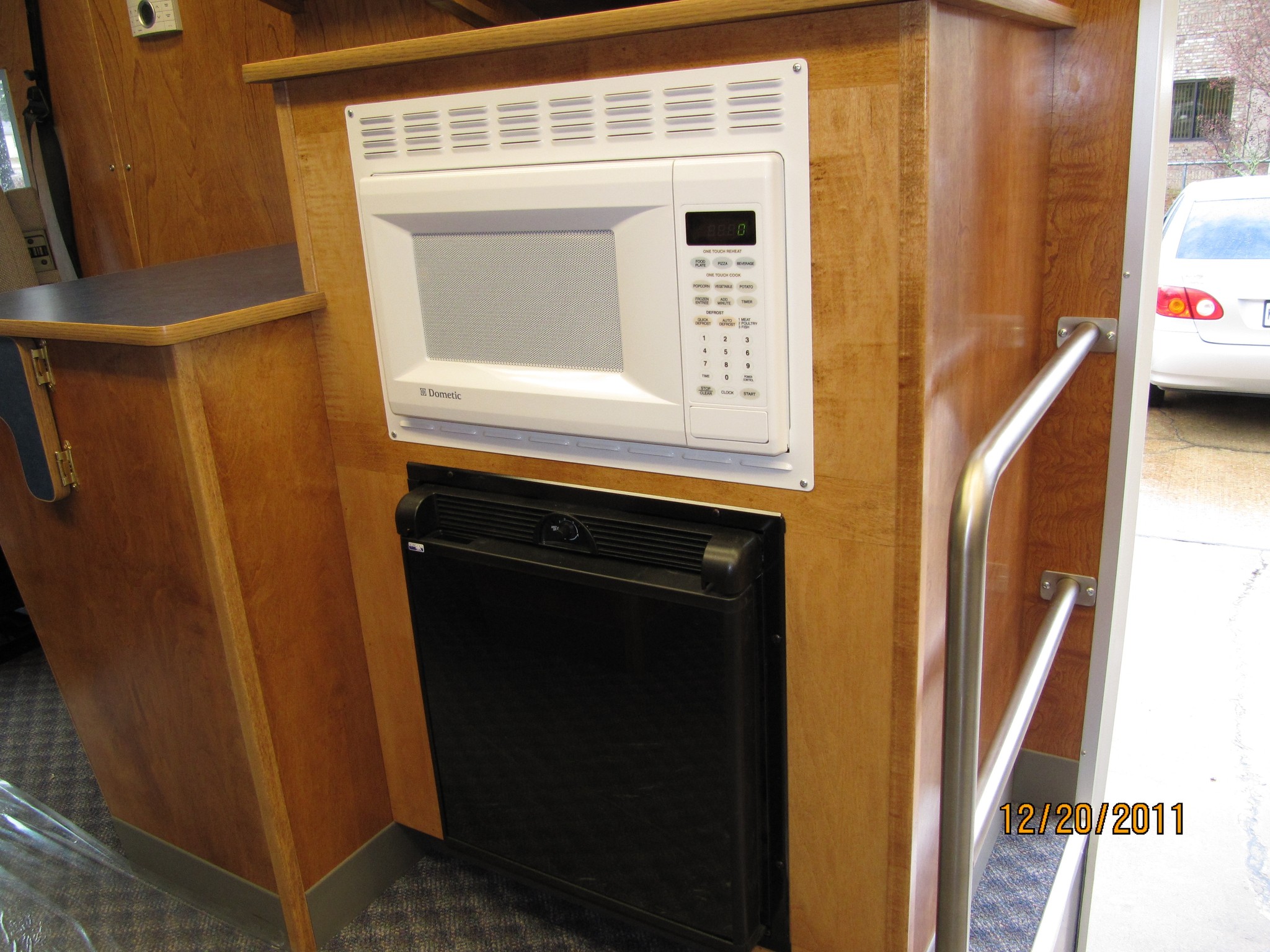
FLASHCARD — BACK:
[358,151,790,455]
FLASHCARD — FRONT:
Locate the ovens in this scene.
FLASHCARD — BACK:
[395,459,791,952]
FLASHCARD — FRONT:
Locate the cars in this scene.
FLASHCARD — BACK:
[1148,176,1270,409]
